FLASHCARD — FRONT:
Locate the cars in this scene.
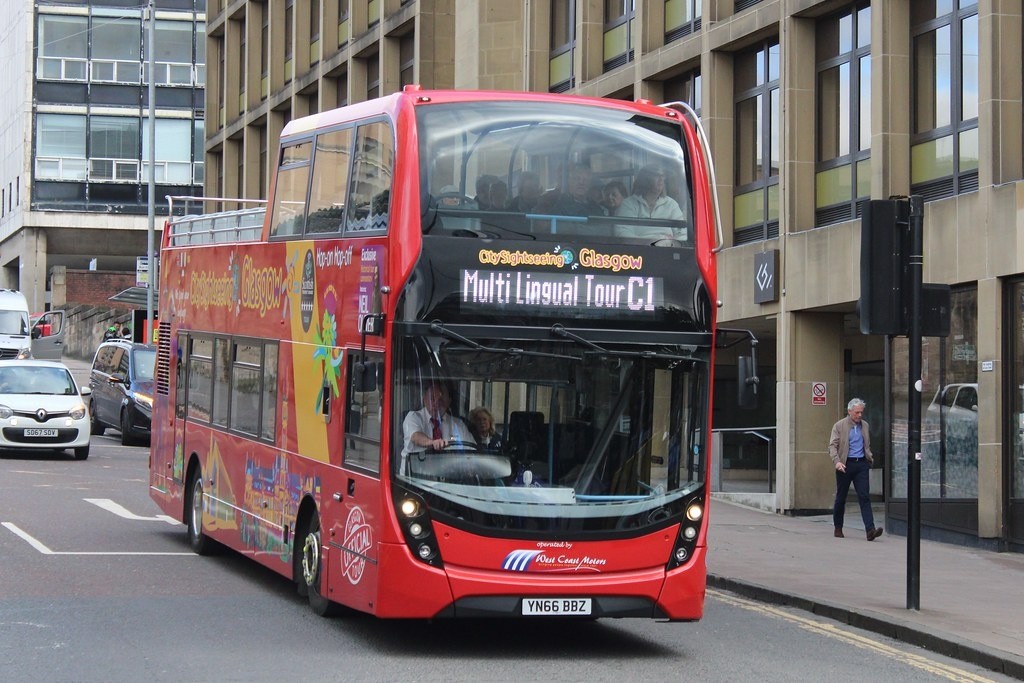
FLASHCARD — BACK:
[0,360,93,459]
[29,312,51,337]
[924,380,1024,467]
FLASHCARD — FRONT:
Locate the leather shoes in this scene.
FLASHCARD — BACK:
[834,527,844,538]
[867,527,883,541]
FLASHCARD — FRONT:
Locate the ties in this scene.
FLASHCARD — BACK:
[430,418,442,439]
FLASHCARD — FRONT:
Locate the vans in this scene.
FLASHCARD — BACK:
[0,287,68,362]
[86,339,159,446]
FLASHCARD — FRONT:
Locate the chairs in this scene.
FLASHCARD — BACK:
[510,412,596,468]
[175,208,266,245]
[436,189,482,231]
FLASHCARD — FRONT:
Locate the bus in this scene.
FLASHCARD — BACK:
[146,83,761,633]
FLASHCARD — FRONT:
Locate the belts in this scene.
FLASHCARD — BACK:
[847,457,866,462]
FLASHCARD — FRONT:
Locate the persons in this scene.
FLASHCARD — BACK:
[828,398,884,541]
[102,321,132,344]
[466,405,503,450]
[400,382,477,478]
[613,163,687,241]
[474,163,628,242]
